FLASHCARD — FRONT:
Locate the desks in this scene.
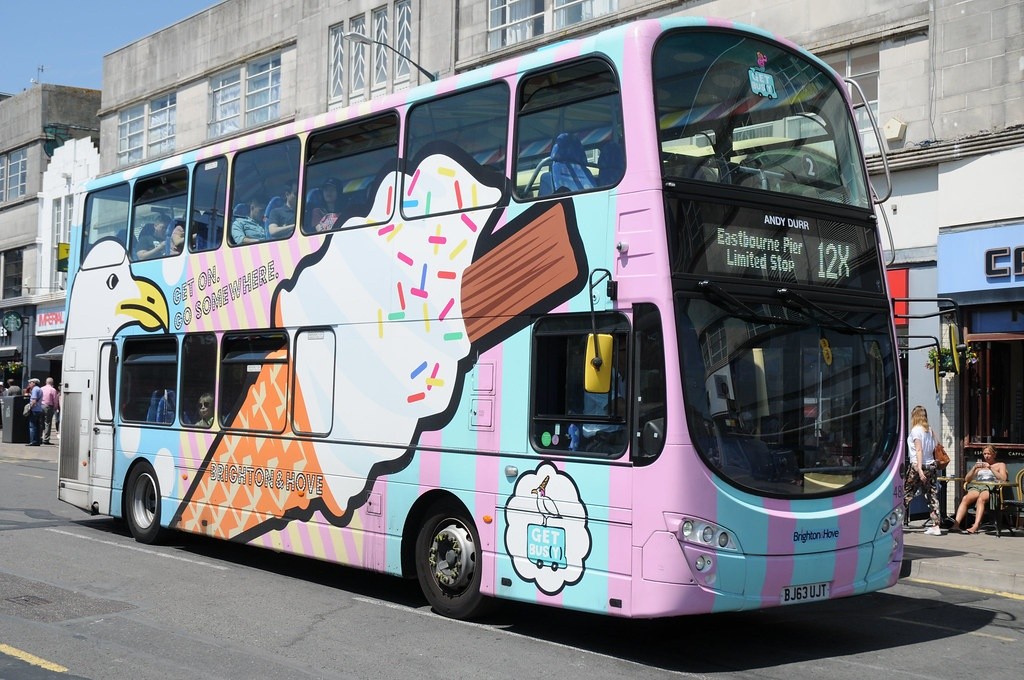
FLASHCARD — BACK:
[971,481,1019,538]
[922,477,964,527]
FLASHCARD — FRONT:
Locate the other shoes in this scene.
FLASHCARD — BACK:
[43,442,55,445]
[25,443,40,446]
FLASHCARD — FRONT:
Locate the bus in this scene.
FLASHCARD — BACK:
[53,17,970,625]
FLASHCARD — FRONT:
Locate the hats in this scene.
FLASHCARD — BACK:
[28,378,40,384]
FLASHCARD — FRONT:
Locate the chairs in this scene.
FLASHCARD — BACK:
[146,390,164,421]
[156,390,190,424]
[230,203,250,244]
[550,133,597,192]
[1015,469,1024,530]
[116,228,138,260]
[264,196,286,240]
[138,223,155,241]
[165,218,184,256]
[195,211,223,251]
[304,188,324,232]
[596,142,624,186]
[964,469,1008,531]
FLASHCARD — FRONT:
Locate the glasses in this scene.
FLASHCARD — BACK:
[199,402,212,409]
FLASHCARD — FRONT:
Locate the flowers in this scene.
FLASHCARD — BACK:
[923,339,980,370]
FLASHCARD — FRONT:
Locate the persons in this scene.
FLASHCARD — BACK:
[135,177,351,260]
[194,393,215,428]
[949,445,1006,533]
[580,333,632,453]
[0,378,62,446]
[904,405,950,536]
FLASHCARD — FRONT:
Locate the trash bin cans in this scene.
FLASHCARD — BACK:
[3,395,32,443]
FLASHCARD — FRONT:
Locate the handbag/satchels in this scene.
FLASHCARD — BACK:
[932,429,950,470]
[23,403,32,417]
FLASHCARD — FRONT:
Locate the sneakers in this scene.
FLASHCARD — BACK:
[924,527,941,536]
